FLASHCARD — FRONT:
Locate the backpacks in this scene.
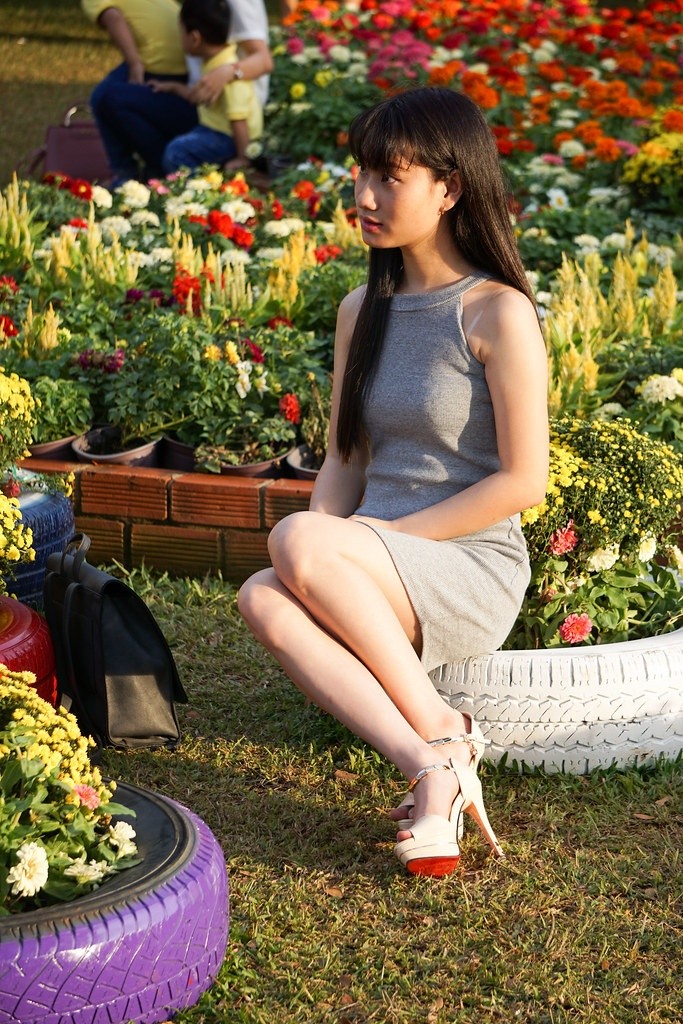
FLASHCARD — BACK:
[42,530,189,756]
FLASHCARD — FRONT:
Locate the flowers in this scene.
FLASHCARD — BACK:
[0,0,683,1024]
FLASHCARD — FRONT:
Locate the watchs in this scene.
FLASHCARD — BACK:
[231,63,243,80]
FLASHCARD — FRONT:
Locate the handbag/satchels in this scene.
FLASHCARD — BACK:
[26,103,112,184]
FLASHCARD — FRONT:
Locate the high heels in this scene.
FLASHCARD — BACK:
[393,757,504,877]
[396,710,492,832]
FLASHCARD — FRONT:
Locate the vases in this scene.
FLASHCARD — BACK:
[158,434,196,471]
[0,595,58,707]
[287,443,321,480]
[0,778,230,1024]
[72,427,162,467]
[0,470,77,612]
[29,433,78,461]
[427,623,683,777]
[220,446,295,478]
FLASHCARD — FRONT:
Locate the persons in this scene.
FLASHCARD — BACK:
[80,0,188,196]
[235,78,548,874]
[145,0,263,178]
[189,0,275,123]
